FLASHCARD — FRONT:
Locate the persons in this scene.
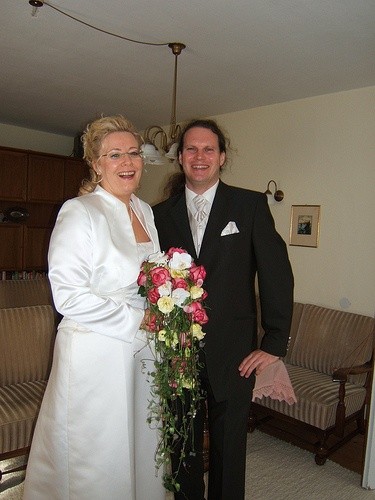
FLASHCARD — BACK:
[151,119,294,500]
[24,115,173,500]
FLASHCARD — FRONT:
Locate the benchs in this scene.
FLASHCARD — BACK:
[0,278,63,460]
[248,302,375,465]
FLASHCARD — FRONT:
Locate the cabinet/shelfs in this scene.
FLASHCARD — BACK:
[0,148,88,205]
[0,223,51,271]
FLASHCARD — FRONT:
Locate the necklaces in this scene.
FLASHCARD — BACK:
[130,207,133,224]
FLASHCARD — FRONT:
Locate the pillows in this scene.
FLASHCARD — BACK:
[1,305,59,386]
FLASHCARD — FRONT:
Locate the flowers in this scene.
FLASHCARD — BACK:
[137,246,212,494]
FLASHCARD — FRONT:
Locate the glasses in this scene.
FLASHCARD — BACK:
[100,149,145,161]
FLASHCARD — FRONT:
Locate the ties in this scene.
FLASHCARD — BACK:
[192,194,209,223]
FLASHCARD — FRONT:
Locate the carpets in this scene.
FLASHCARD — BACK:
[245,427,375,500]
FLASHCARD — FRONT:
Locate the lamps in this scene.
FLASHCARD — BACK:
[142,43,185,164]
[264,180,284,202]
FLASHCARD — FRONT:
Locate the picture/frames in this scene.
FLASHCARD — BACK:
[289,205,320,248]
